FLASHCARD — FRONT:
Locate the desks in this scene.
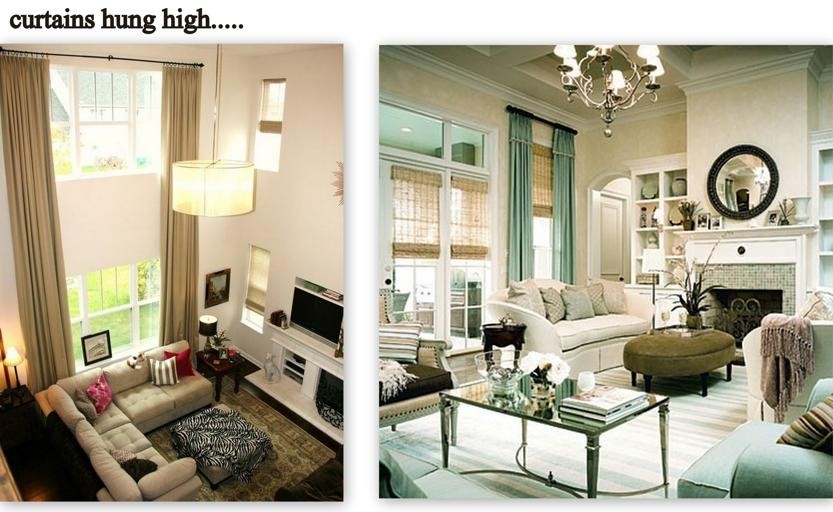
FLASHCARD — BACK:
[0,385,35,448]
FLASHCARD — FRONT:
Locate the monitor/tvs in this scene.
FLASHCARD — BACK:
[289,285,344,348]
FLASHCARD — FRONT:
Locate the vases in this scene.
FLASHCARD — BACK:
[678,199,703,231]
[790,196,812,225]
[686,313,703,328]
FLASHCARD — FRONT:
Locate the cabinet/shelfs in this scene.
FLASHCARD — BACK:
[244,320,343,444]
[808,129,833,293]
[625,151,687,284]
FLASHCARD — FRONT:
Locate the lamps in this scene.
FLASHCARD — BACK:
[171,43,256,218]
[552,45,665,140]
[198,314,218,358]
[3,347,24,387]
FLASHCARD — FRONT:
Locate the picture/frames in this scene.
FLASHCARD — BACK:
[205,268,231,308]
[80,329,112,367]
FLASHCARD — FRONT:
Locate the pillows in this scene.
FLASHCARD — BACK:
[796,288,833,321]
[775,392,833,450]
[379,322,423,365]
[46,340,198,502]
[506,277,627,324]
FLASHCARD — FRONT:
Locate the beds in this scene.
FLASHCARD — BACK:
[624,329,736,397]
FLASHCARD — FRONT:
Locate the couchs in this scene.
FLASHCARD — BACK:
[34,340,213,502]
[486,279,657,373]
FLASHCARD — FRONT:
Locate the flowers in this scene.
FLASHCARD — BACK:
[648,230,726,315]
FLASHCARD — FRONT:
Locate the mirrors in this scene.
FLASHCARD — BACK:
[706,144,779,219]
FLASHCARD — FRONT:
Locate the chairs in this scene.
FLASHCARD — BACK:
[677,377,833,498]
[378,288,458,446]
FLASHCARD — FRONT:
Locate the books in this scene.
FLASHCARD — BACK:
[558,385,649,427]
